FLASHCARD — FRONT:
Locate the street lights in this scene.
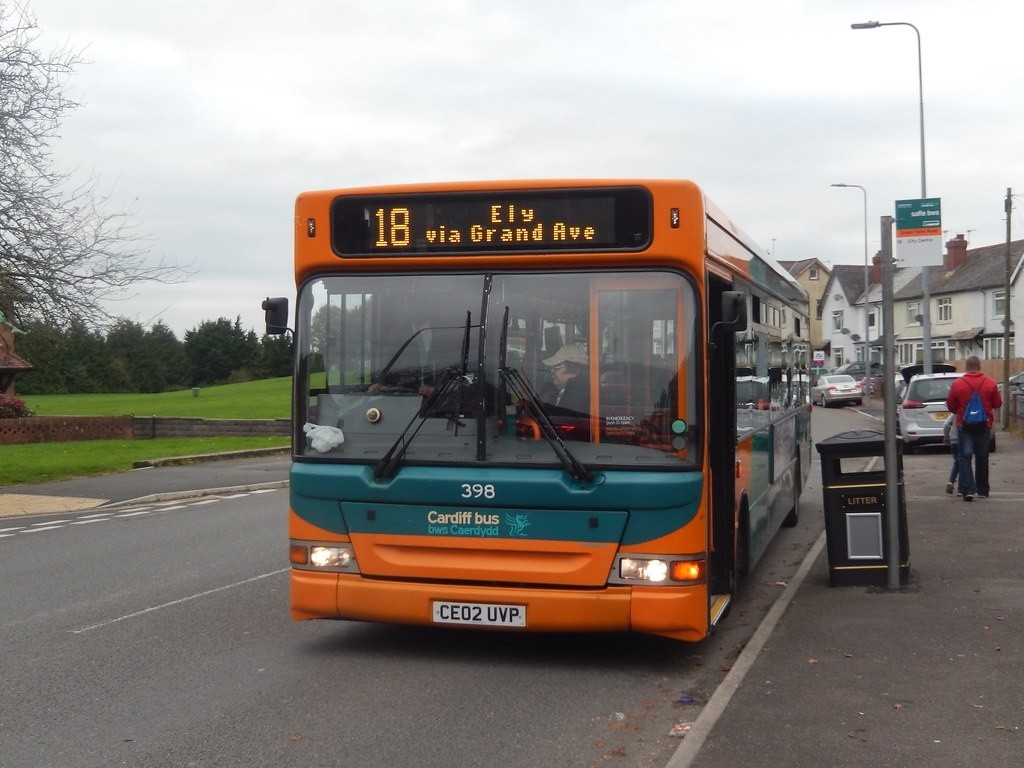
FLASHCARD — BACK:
[849,21,931,376]
[831,183,871,406]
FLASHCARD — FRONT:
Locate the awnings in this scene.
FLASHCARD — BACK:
[869,335,898,352]
[815,339,830,357]
[949,327,984,351]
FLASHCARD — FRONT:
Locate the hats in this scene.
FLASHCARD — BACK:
[542,343,589,367]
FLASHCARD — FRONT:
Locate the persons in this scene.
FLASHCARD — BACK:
[946,356,1002,500]
[542,344,589,417]
[943,413,963,497]
[368,291,453,397]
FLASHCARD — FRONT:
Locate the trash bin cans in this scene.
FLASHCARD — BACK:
[814,430,911,588]
[192,387,200,397]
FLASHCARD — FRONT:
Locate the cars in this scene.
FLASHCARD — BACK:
[814,374,864,405]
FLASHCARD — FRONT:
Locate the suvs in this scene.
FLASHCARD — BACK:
[896,372,995,455]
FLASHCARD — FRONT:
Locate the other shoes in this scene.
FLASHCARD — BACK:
[976,490,989,498]
[945,479,954,494]
[957,490,964,496]
[963,493,974,501]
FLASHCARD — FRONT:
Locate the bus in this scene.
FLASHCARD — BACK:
[261,178,812,644]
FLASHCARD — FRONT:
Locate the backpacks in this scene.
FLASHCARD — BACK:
[958,377,988,433]
